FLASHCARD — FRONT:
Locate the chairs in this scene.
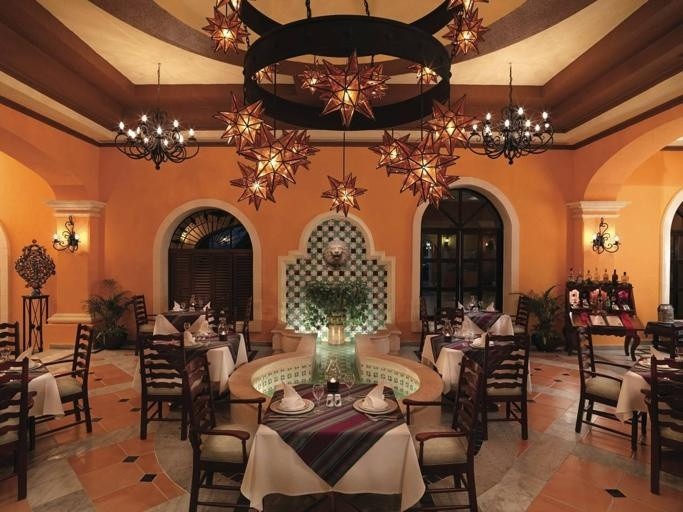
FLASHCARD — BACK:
[402,354,487,511]
[135,326,188,441]
[419,296,533,358]
[135,296,254,352]
[463,330,530,440]
[0,321,94,502]
[575,321,683,495]
[174,348,267,510]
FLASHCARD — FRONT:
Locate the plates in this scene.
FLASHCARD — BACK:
[637,345,677,372]
[175,342,204,350]
[269,398,316,416]
[9,362,44,371]
[351,397,398,416]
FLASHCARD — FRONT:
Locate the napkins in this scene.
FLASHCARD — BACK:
[277,380,306,411]
[358,382,389,410]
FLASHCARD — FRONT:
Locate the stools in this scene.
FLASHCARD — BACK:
[44,313,92,353]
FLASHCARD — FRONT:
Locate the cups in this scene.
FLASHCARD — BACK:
[440,294,496,349]
[183,323,190,330]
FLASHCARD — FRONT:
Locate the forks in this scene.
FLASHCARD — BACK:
[363,411,398,422]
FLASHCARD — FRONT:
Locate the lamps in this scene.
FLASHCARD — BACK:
[468,62,555,165]
[113,63,200,170]
[202,0,491,218]
[592,217,621,254]
[51,215,80,253]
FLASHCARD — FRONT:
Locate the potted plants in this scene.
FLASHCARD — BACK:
[303,279,366,346]
[81,279,140,349]
[13,239,56,296]
[510,284,564,352]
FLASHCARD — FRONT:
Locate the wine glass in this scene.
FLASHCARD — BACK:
[343,373,357,402]
[313,384,325,415]
[197,300,203,313]
[1,346,12,362]
[180,303,185,314]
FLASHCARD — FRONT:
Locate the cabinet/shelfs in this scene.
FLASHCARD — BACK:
[564,281,646,361]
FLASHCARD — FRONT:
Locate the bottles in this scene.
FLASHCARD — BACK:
[566,267,628,286]
[581,287,630,311]
[324,357,340,392]
[325,394,336,408]
[333,394,342,408]
[216,320,228,340]
[188,295,196,307]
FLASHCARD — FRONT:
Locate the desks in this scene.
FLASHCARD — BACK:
[21,296,50,352]
